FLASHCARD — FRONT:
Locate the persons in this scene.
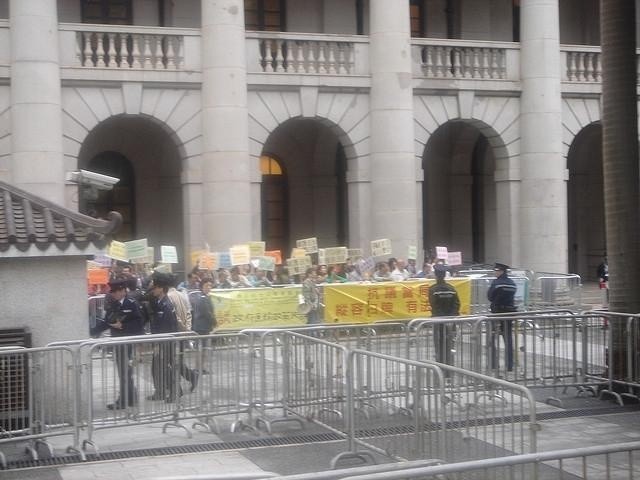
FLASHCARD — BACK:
[429,263,461,389]
[487,259,519,372]
[142,278,183,405]
[303,268,320,344]
[192,278,217,375]
[89,280,143,411]
[87,255,471,344]
[597,251,609,303]
[163,273,200,392]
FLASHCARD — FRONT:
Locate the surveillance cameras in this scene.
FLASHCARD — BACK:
[77,169,120,190]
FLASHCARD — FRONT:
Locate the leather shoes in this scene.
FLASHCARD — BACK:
[190,368,200,392]
[146,390,165,401]
[106,397,137,409]
[165,388,183,403]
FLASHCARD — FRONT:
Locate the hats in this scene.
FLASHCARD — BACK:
[432,264,449,272]
[491,263,509,271]
[106,278,128,292]
[152,277,169,288]
